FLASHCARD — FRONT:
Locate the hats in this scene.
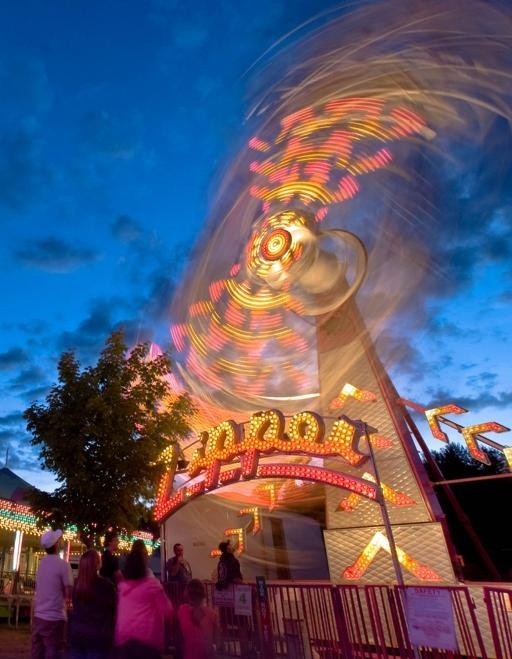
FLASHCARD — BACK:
[218,539,231,552]
[40,527,63,550]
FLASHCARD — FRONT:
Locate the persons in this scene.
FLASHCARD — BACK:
[30,528,74,659]
[176,579,219,658]
[213,540,244,590]
[130,538,154,577]
[115,551,175,658]
[68,549,117,659]
[100,533,121,578]
[167,543,193,582]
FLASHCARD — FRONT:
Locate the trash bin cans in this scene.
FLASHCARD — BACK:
[282,618,312,659]
